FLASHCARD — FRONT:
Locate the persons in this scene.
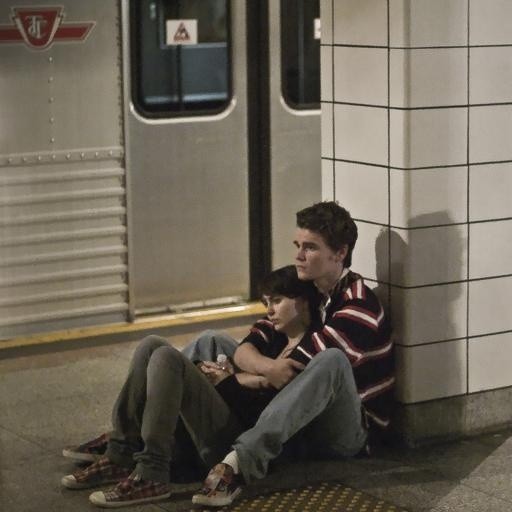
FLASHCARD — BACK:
[61,201,398,510]
[58,261,324,511]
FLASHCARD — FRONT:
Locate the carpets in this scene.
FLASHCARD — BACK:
[203,478,412,512]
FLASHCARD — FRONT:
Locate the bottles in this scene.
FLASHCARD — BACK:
[216,354,235,379]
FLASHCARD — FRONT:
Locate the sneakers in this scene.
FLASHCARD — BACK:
[61,429,111,460]
[191,462,247,506]
[60,459,134,491]
[85,476,173,508]
